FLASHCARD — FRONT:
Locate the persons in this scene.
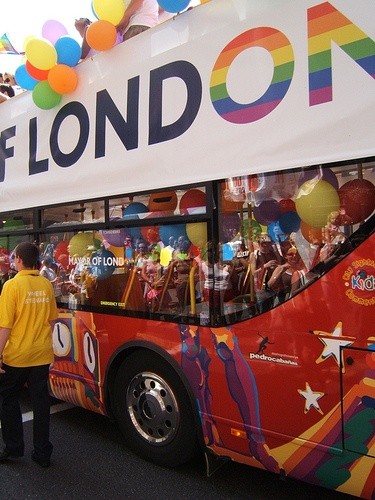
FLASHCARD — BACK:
[0,242,59,468]
[4,72,26,104]
[115,0,159,42]
[0,84,15,104]
[0,208,354,332]
[0,73,4,85]
[75,17,99,62]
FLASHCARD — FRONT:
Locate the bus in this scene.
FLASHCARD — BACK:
[0,0,375,500]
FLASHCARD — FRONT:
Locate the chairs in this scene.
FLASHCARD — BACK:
[92,252,251,315]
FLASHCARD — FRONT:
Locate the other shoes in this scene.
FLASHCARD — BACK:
[31,450,50,467]
[0,449,25,463]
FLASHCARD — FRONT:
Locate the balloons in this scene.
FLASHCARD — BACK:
[0,166,375,275]
[0,0,214,110]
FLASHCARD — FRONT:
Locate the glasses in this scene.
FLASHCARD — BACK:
[287,253,298,256]
[12,255,22,259]
